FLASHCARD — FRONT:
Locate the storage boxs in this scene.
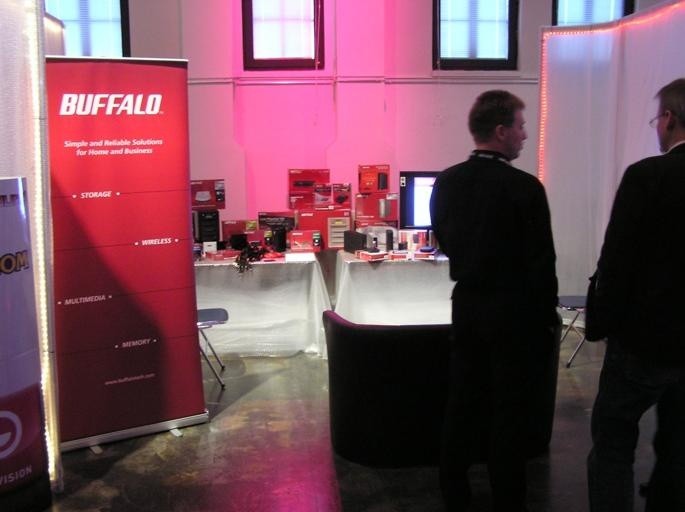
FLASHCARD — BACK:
[191,164,400,253]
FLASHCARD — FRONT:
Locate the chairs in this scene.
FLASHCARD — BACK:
[194,308,230,388]
[557,295,588,367]
[322,309,462,469]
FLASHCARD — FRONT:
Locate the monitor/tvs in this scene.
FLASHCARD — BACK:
[399,171,440,239]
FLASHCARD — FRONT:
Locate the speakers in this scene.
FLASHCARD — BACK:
[193,210,219,242]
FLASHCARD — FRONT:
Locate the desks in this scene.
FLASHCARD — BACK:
[337,250,458,325]
[194,250,329,358]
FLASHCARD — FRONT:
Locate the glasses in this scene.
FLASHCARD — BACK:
[649,113,668,128]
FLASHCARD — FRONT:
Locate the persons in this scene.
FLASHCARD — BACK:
[429,90,562,512]
[584,77,685,510]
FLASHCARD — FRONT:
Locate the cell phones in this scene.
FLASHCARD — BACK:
[421,246,435,252]
